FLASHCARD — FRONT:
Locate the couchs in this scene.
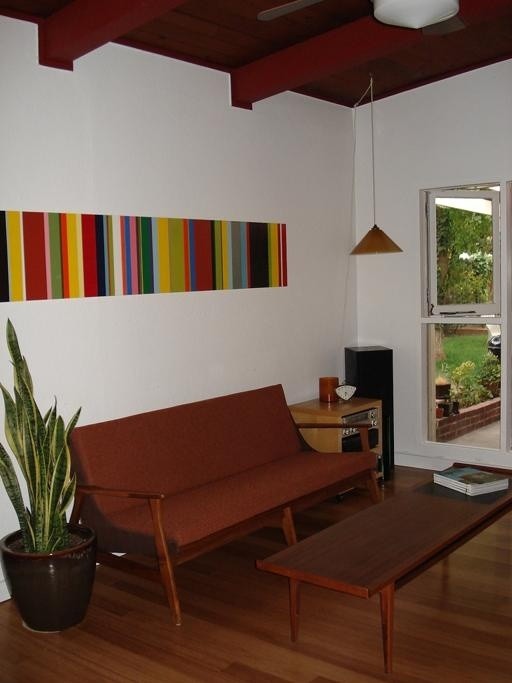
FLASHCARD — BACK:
[67,383,383,631]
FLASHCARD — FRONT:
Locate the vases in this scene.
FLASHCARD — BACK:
[438,403,450,416]
[437,407,446,417]
[453,401,460,413]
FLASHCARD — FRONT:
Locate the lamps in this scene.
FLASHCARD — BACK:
[352,72,404,255]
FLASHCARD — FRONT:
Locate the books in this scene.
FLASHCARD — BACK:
[433,465,509,497]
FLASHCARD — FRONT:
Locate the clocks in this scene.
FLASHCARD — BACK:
[335,384,357,402]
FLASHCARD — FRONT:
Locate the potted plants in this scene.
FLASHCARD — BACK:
[478,352,501,396]
[436,377,452,398]
[0,318,99,632]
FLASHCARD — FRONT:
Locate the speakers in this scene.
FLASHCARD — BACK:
[344,345,394,481]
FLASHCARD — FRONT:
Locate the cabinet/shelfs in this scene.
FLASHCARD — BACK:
[287,392,386,489]
